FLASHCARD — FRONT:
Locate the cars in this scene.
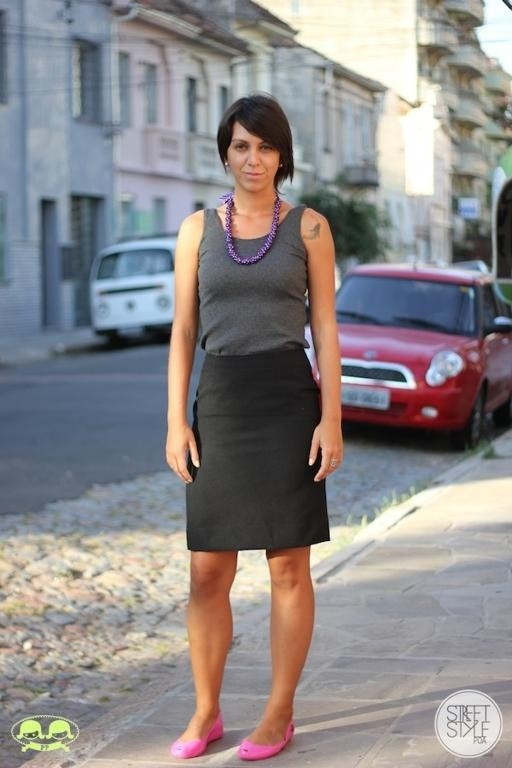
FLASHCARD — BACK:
[302,261,512,449]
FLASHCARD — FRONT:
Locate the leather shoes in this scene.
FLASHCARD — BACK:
[237,717,297,760]
[169,712,225,761]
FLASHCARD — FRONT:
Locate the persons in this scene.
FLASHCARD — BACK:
[164,94,345,760]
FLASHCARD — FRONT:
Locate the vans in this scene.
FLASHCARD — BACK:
[89,235,179,344]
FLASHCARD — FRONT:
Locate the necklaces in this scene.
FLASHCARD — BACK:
[219,192,280,265]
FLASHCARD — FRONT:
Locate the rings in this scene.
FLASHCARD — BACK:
[329,456,338,469]
